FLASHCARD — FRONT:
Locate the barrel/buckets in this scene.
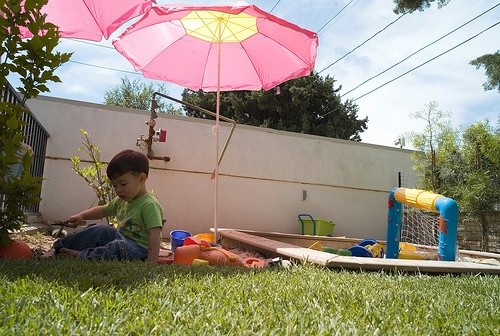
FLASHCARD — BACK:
[170,230,192,253]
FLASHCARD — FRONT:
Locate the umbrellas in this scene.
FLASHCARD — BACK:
[0,0,157,42]
[111,0,319,245]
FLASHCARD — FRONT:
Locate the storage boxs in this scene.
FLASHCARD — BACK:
[297,218,335,236]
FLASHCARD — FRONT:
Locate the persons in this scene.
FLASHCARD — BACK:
[37,149,162,266]
